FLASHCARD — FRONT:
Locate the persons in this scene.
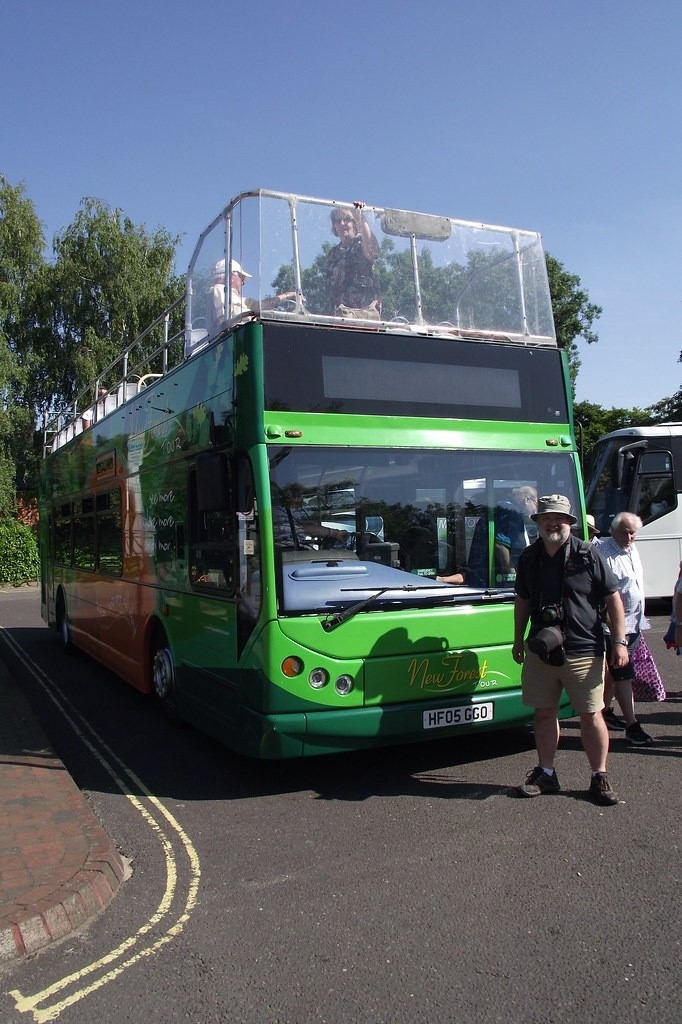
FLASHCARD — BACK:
[206,259,307,337]
[511,494,629,806]
[272,482,349,551]
[326,201,382,322]
[594,512,653,744]
[663,560,682,656]
[467,485,538,575]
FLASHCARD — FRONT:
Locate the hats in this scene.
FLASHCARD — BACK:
[586,514,600,534]
[214,258,254,279]
[531,494,578,526]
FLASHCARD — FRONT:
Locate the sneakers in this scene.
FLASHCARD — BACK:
[625,721,653,745]
[588,771,617,806]
[520,766,561,796]
[601,706,626,730]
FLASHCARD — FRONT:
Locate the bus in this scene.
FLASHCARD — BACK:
[578,420,682,600]
[32,190,592,763]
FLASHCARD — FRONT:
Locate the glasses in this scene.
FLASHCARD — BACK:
[332,217,353,224]
[237,274,245,285]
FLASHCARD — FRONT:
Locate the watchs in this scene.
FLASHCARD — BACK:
[613,639,628,646]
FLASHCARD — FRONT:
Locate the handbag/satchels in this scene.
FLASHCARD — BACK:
[615,632,666,703]
[336,299,383,333]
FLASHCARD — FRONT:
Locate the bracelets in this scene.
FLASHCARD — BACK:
[676,622,682,625]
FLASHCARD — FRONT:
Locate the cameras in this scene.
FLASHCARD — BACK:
[540,603,564,622]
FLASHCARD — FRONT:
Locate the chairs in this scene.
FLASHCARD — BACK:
[189,315,210,354]
[433,319,464,341]
[51,363,163,452]
[384,315,412,336]
[271,299,306,314]
